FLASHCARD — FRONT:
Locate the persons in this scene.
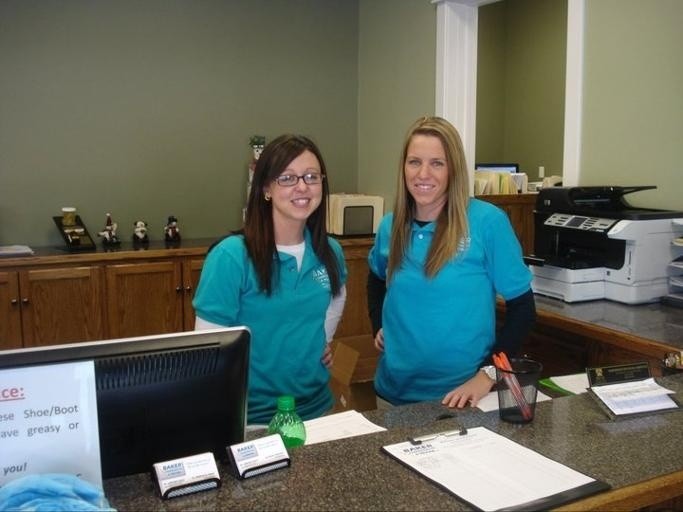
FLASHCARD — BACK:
[366,116,537,409]
[192,133,348,431]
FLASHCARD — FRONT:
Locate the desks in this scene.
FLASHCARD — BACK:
[103,373,682,511]
[475,194,536,257]
[497,294,683,372]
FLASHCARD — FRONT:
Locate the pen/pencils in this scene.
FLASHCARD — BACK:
[492,351,532,421]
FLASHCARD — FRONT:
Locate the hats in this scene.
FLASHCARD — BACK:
[134,220,146,227]
[250,135,265,147]
[167,216,177,225]
[105,213,113,226]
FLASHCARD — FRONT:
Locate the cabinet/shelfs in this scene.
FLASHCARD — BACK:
[1,240,370,348]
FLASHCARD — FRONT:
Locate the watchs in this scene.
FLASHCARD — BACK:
[480,364,502,383]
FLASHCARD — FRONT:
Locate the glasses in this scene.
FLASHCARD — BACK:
[263,172,326,186]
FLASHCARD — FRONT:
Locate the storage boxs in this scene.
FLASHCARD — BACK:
[328,333,379,412]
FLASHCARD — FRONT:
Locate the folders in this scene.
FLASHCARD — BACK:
[380,424,611,511]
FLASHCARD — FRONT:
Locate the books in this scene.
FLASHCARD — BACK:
[473,170,528,196]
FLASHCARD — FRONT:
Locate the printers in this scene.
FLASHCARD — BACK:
[524,184,683,306]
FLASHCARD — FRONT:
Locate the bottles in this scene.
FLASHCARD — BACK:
[267,395,307,450]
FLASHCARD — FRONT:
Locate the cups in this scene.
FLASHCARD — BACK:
[496,357,543,424]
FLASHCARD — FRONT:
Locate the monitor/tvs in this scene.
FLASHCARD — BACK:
[474,162,520,174]
[0,326,253,481]
